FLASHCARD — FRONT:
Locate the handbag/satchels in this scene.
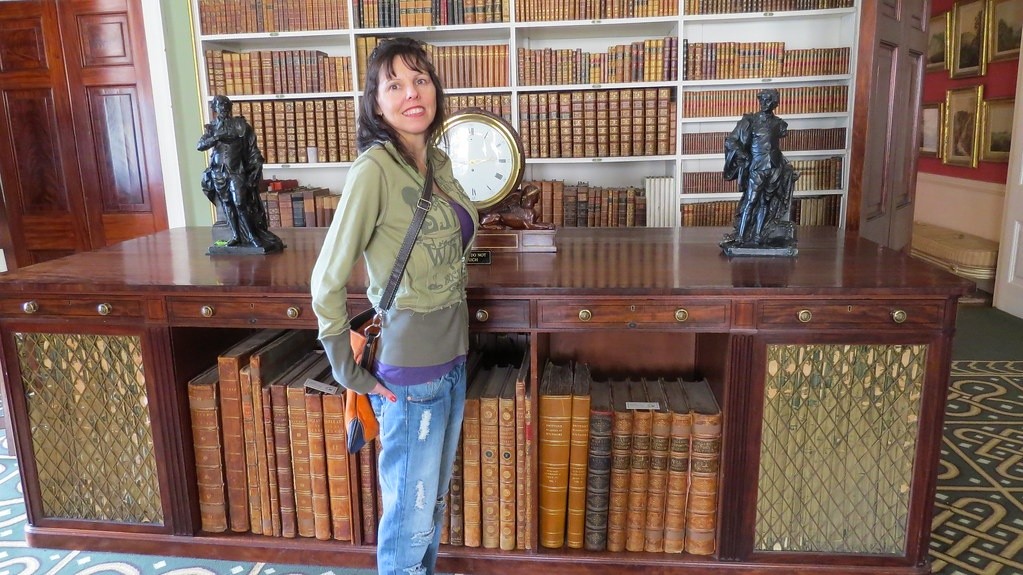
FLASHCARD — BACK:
[344,308,382,456]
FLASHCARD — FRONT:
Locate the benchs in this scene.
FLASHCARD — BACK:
[911,220,999,304]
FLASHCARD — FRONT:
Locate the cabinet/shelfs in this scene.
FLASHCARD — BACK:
[188,0,863,228]
[0,226,978,575]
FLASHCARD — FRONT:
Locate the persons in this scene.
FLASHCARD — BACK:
[717,88,801,247]
[196,95,282,248]
[309,35,480,575]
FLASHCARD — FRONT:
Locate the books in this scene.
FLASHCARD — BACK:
[188,328,721,555]
[198,0,854,227]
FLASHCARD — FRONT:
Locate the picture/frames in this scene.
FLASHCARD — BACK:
[978,99,1015,162]
[942,84,984,168]
[925,12,950,73]
[948,0,989,79]
[918,102,943,159]
[987,0,1023,64]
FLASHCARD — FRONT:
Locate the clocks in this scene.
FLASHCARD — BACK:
[433,106,556,231]
